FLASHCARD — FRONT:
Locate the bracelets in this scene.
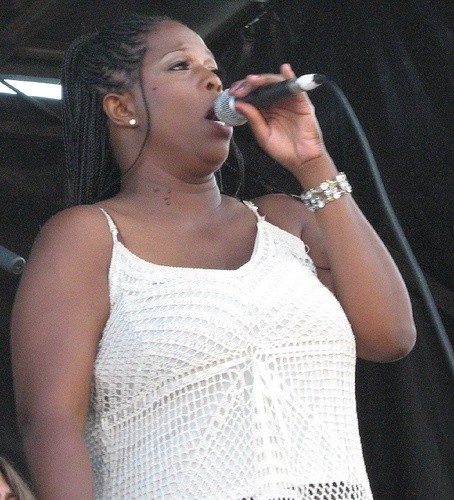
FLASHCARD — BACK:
[301,172,352,213]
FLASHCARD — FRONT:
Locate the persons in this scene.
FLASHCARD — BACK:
[9,15,417,500]
[0,456,35,500]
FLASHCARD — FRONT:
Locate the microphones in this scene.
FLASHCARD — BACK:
[214,73,327,127]
